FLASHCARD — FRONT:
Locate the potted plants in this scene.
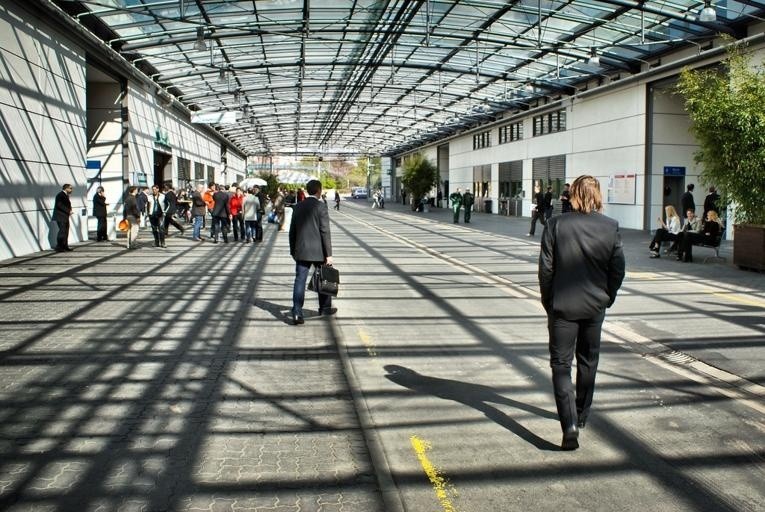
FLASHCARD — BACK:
[657,29,765,273]
[400,152,441,211]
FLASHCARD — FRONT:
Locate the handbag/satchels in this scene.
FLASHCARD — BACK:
[268,211,280,224]
[307,264,340,297]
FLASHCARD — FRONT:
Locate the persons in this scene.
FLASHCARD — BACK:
[558,182,571,214]
[437,188,442,207]
[681,183,696,226]
[289,179,338,325]
[124,182,305,247]
[664,208,703,262]
[525,185,548,236]
[49,183,75,252]
[544,185,554,222]
[450,188,474,224]
[683,211,726,263]
[322,191,340,209]
[372,189,384,209]
[91,186,113,244]
[402,190,406,206]
[702,185,722,225]
[536,175,626,452]
[648,205,682,259]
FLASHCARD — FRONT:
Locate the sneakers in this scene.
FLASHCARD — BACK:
[649,249,683,260]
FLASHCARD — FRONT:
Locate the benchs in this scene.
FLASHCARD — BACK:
[665,226,726,262]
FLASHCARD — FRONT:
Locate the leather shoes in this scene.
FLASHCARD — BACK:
[294,315,304,326]
[162,243,168,248]
[562,426,581,451]
[579,422,585,428]
[192,234,261,243]
[319,307,337,316]
[152,242,158,246]
[56,247,73,251]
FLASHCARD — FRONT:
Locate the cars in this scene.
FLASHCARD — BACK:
[351,186,368,199]
